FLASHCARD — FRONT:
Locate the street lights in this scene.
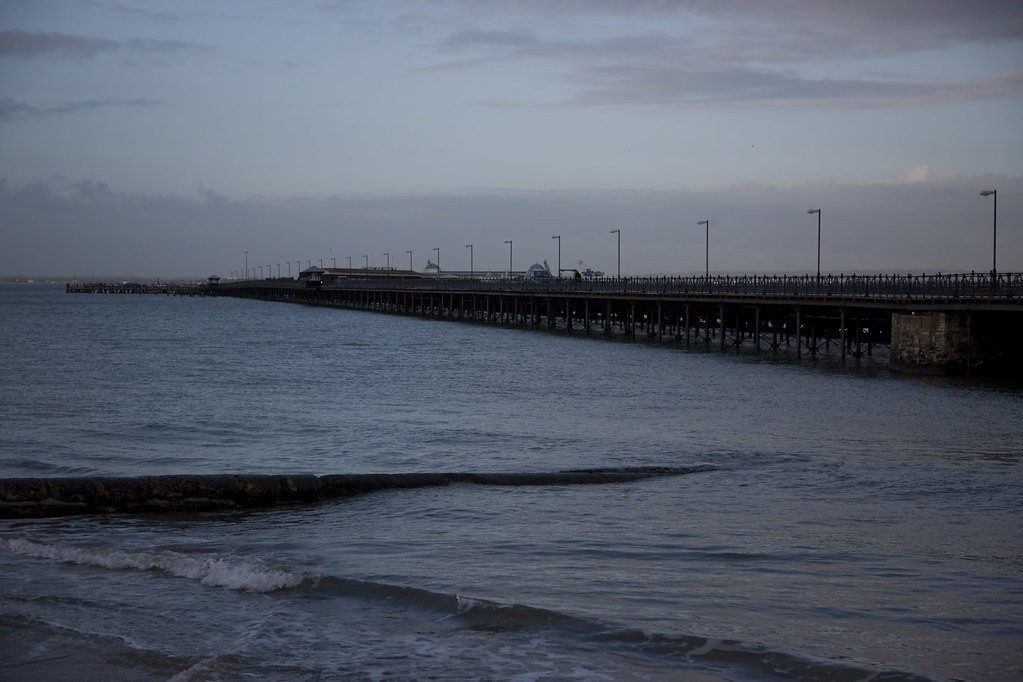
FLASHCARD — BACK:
[433,247,440,277]
[504,240,513,279]
[697,220,708,280]
[551,235,562,278]
[610,229,620,281]
[319,254,369,283]
[979,188,997,281]
[806,208,821,286]
[382,252,391,280]
[248,260,311,281]
[405,250,414,276]
[465,243,474,279]
